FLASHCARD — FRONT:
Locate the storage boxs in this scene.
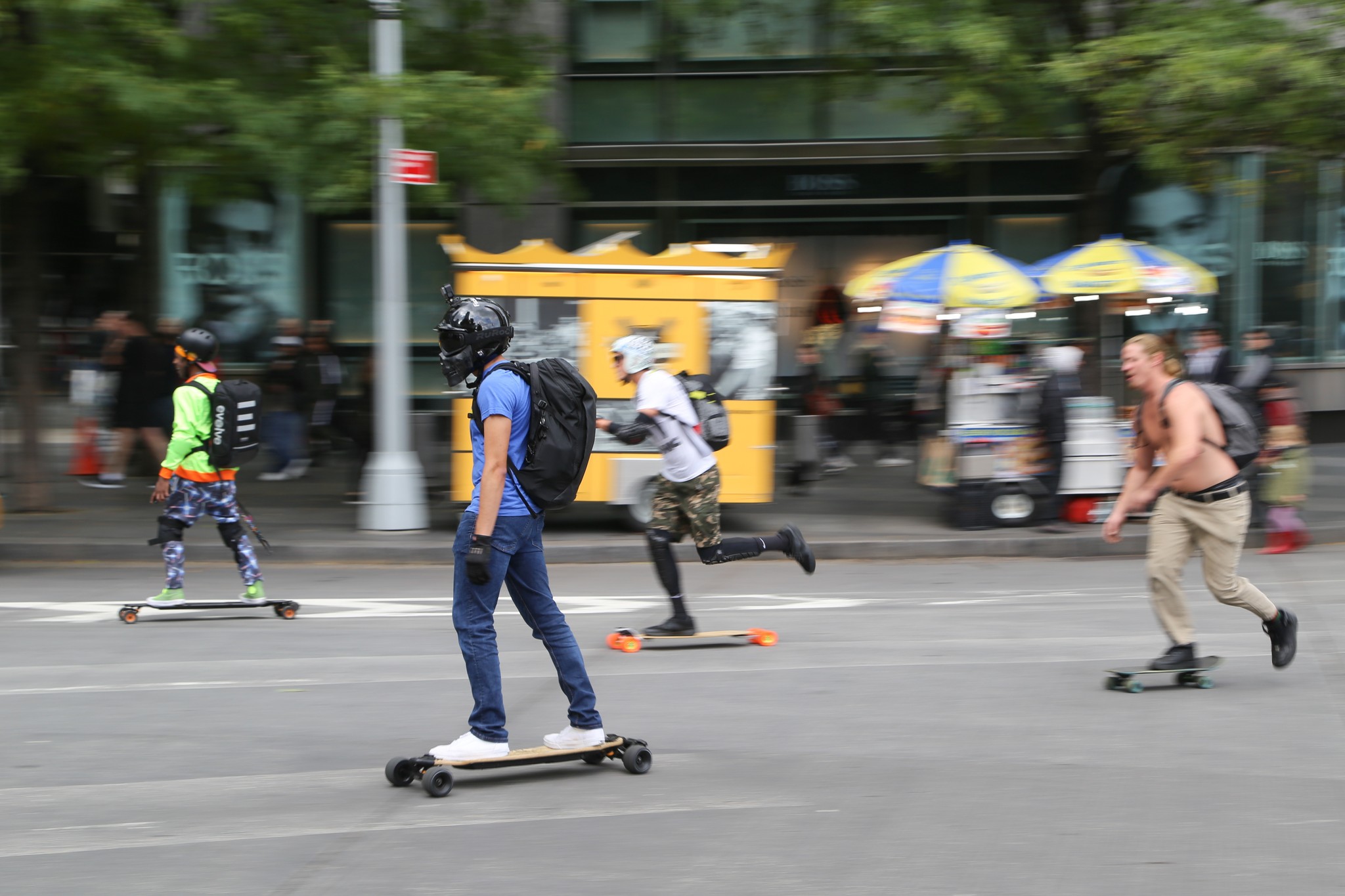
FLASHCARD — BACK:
[68,370,121,404]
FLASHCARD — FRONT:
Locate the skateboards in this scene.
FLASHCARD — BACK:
[607,627,779,652]
[119,600,299,623]
[386,735,652,797]
[1101,654,1220,693]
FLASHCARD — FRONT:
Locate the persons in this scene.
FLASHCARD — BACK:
[1090,334,1297,669]
[81,304,170,490]
[594,336,815,636]
[1235,328,1313,554]
[429,297,606,762]
[145,327,264,601]
[264,316,347,483]
[711,308,780,401]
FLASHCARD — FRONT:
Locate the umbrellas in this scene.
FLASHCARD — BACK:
[841,243,1049,338]
[1028,237,1219,318]
